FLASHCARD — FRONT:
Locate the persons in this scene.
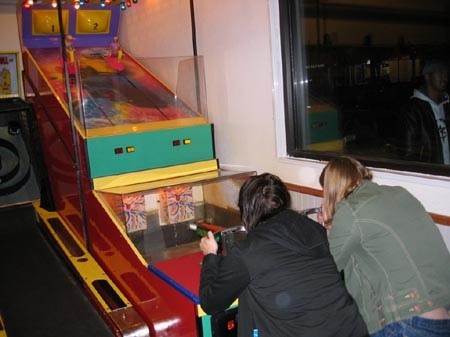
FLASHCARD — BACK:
[199,173,371,337]
[396,59,450,164]
[317,156,450,337]
